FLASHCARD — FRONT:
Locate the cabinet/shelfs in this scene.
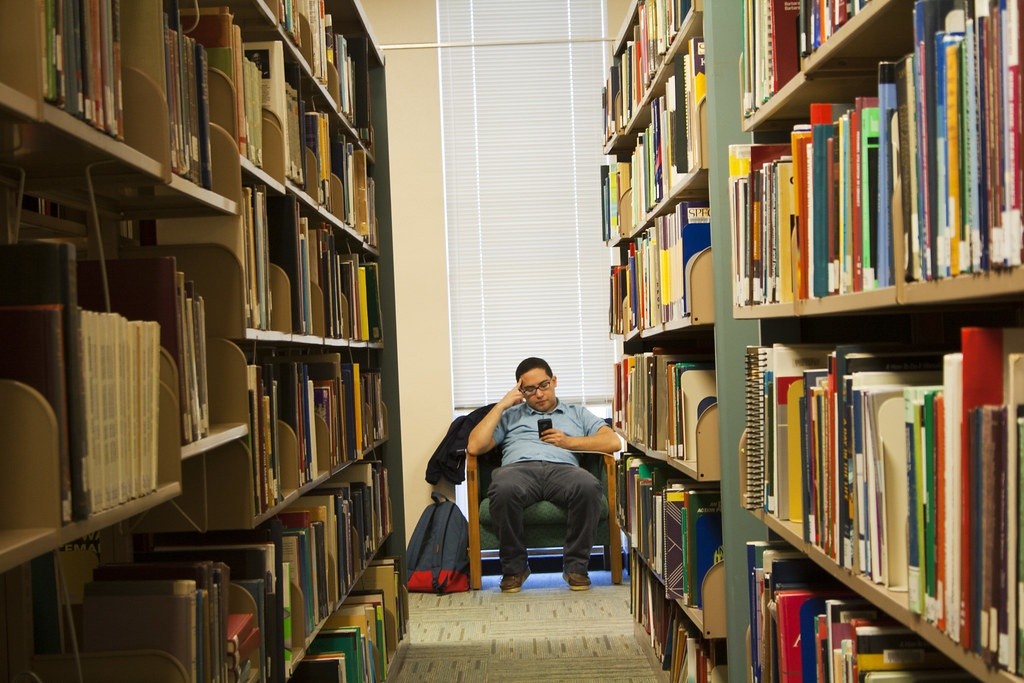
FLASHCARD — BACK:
[602,0,1024,683]
[0,0,411,683]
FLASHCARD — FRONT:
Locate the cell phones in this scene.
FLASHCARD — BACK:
[538,418,553,440]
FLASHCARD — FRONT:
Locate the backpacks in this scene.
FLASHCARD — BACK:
[407,491,469,593]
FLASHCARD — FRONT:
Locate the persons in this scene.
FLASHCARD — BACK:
[468,358,621,591]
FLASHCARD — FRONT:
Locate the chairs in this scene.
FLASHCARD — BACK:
[466,443,623,590]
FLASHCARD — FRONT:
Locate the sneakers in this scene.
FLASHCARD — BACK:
[500,565,531,592]
[563,570,591,590]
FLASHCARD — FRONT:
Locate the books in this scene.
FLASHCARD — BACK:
[0,1,403,683]
[615,0,1024,683]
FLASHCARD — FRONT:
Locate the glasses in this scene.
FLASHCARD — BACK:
[520,377,551,396]
